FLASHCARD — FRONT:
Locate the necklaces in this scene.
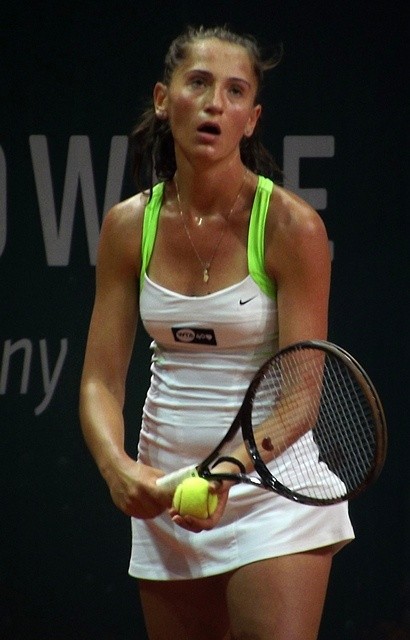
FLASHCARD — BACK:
[172,170,253,282]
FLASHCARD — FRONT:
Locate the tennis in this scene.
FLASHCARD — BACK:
[172,476,218,518]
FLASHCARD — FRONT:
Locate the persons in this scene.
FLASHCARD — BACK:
[79,27,355,638]
